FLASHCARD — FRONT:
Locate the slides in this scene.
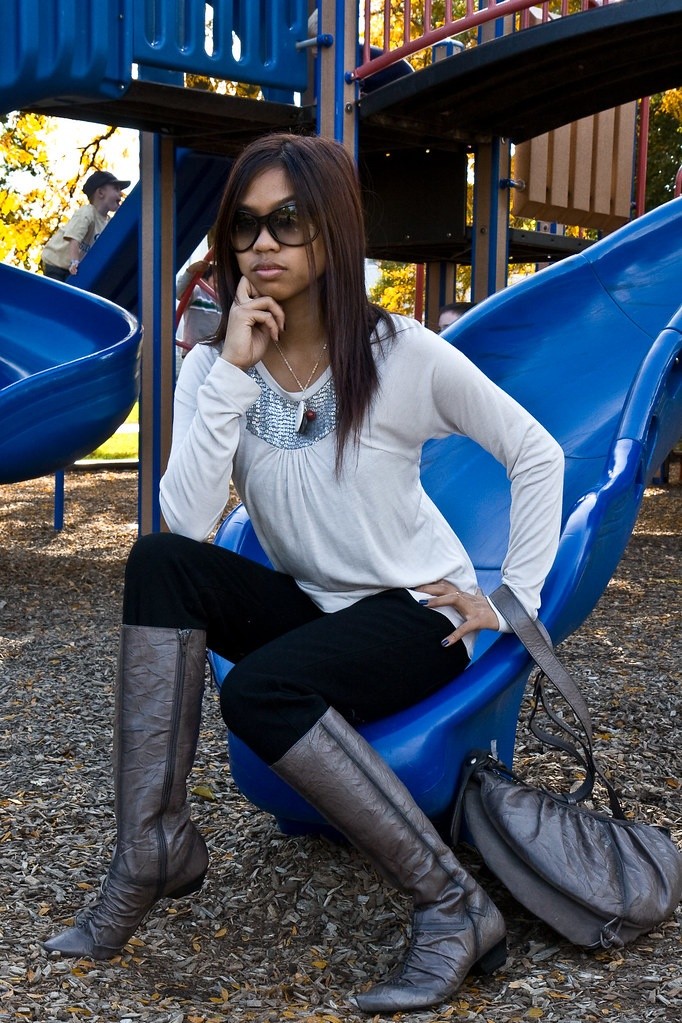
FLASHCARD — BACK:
[203,194,680,843]
[48,136,250,324]
[0,257,140,481]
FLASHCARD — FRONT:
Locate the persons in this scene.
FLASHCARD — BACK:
[307,8,415,105]
[41,132,566,1017]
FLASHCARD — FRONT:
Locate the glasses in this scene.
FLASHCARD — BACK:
[226,202,320,252]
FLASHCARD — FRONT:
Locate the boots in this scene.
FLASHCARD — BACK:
[41,623,208,960]
[269,706,511,1016]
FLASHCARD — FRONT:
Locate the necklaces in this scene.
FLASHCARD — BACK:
[271,339,329,434]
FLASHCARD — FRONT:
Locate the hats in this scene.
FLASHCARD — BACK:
[82,171,131,198]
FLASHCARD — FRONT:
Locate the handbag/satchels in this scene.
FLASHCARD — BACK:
[449,751,682,950]
